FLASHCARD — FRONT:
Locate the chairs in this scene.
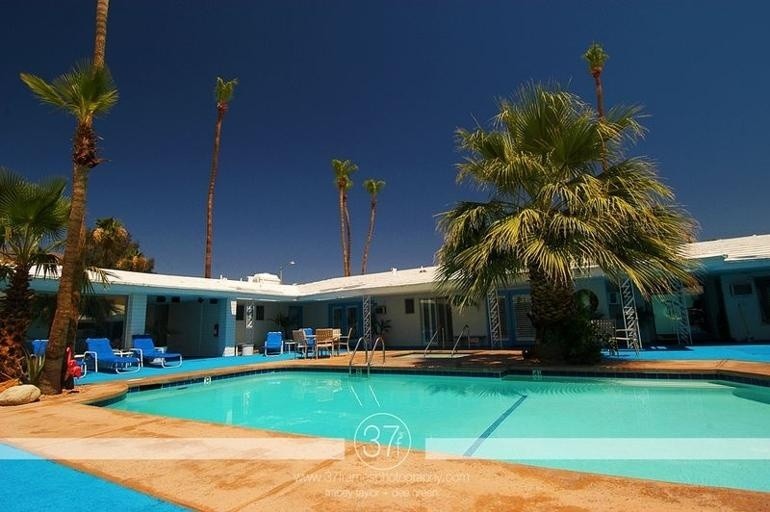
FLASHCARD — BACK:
[30,332,184,384]
[263,327,355,361]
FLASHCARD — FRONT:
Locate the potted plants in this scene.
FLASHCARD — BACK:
[371,315,393,350]
